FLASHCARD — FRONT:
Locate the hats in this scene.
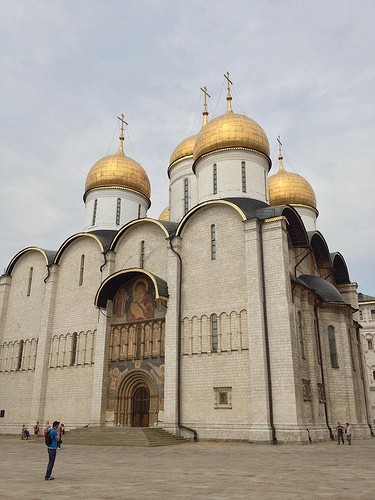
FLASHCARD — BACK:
[52,420,60,425]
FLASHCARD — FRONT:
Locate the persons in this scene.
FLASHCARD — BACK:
[45,421,62,480]
[21,422,65,441]
[336,422,344,444]
[344,423,352,445]
[57,426,62,449]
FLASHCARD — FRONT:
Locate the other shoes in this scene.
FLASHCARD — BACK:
[58,448,61,450]
[45,476,54,480]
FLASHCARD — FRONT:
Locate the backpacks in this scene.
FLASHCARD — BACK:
[44,428,56,447]
